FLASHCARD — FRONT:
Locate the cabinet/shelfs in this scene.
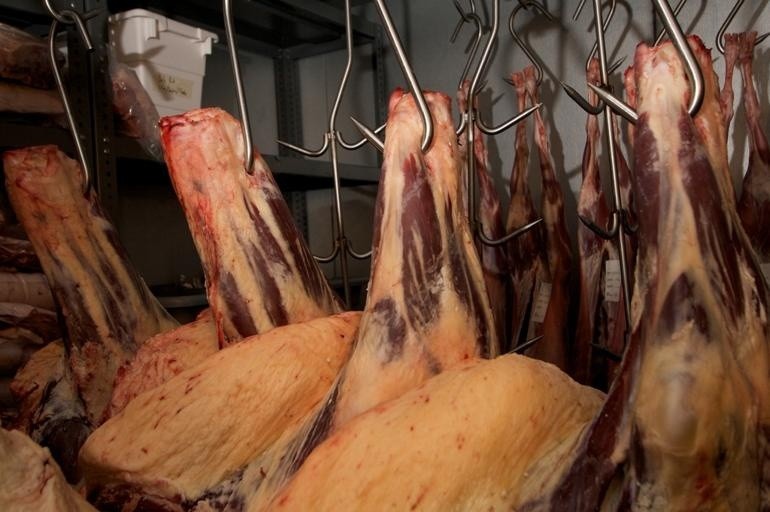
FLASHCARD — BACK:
[67,4,387,314]
